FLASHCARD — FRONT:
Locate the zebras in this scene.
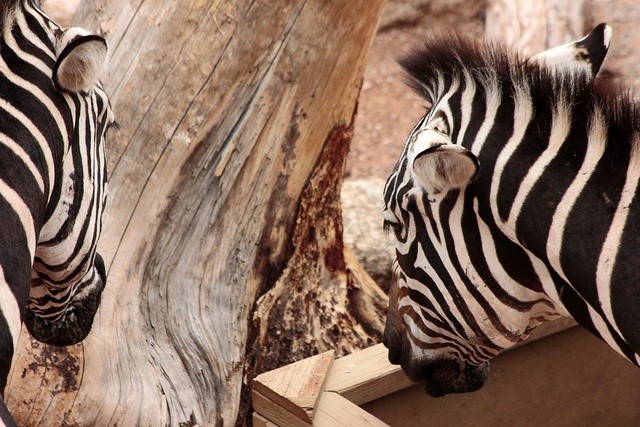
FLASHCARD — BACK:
[0,0,121,425]
[379,21,640,399]
